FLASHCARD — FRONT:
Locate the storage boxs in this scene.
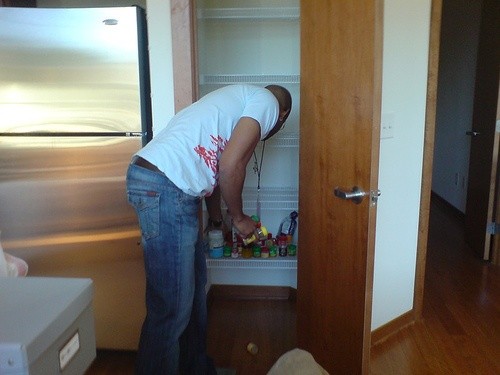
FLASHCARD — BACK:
[0,274,97,375]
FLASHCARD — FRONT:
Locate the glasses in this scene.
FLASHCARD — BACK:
[278,116,286,129]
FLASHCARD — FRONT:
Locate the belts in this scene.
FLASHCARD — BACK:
[130,155,166,176]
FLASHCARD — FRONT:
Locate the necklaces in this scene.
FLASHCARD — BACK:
[251,139,267,192]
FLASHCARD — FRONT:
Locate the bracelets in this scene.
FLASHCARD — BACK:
[208,215,224,227]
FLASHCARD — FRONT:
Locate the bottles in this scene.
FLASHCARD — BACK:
[208,211,298,258]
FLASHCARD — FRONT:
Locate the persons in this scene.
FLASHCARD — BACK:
[126,83,293,375]
